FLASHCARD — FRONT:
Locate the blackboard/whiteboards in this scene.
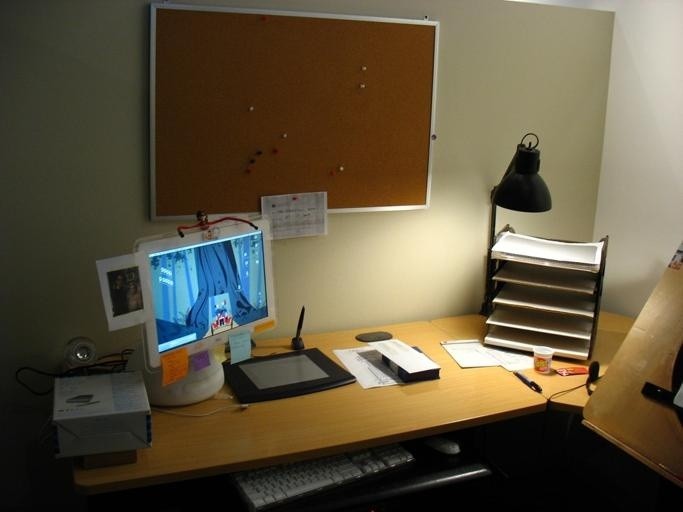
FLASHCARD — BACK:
[149,4,439,221]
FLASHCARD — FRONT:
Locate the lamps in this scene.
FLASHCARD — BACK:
[480,132,553,315]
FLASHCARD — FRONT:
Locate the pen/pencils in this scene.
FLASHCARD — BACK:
[513,370,542,392]
[290,306,304,350]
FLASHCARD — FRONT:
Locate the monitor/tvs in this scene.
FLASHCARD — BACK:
[123,213,278,407]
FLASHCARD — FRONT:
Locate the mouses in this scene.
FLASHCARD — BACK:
[432,438,460,455]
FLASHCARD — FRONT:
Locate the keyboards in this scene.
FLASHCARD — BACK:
[233,443,416,511]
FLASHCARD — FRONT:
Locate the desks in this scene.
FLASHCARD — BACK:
[437,306,635,418]
[73,322,548,512]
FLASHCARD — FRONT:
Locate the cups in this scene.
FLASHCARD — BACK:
[533,346,554,373]
